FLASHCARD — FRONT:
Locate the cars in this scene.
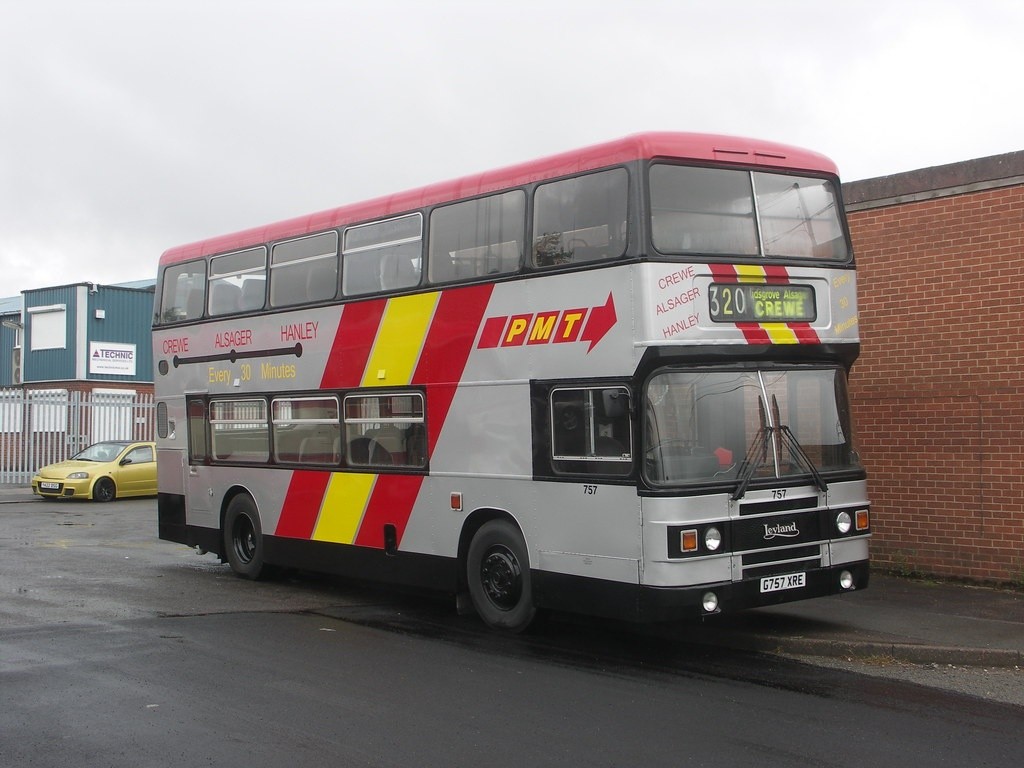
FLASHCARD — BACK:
[31,438,162,503]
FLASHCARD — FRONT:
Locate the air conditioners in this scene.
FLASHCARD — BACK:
[12,348,21,385]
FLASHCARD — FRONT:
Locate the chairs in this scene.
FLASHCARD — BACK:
[186,251,518,320]
[230,424,426,468]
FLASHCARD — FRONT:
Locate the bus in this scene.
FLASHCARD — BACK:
[152,126,875,641]
